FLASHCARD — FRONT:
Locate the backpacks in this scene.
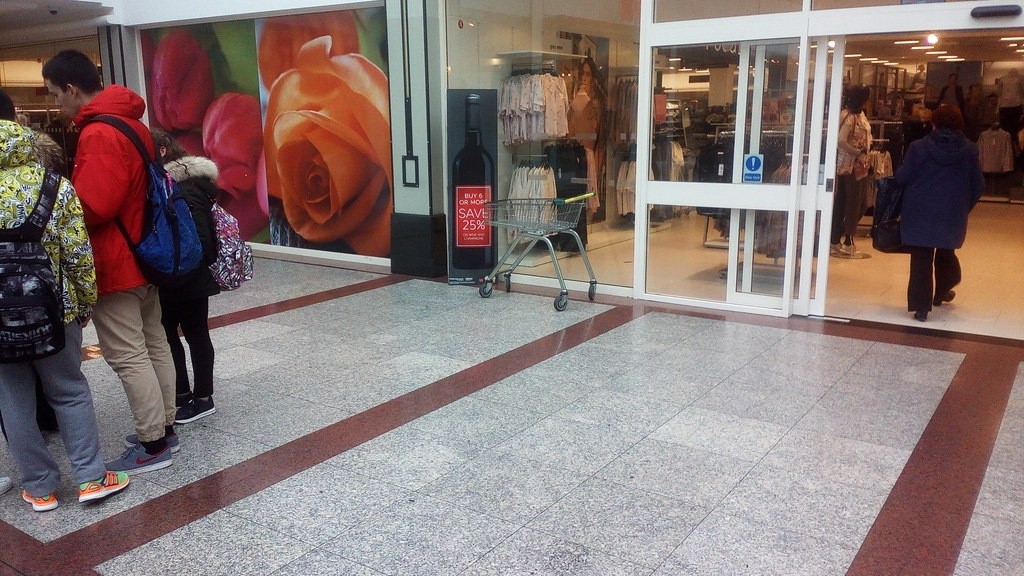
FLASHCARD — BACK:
[0,171,66,362]
[83,115,203,281]
[194,182,253,289]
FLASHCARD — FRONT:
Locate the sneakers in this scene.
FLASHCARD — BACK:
[79,470,129,503]
[125,430,180,455]
[23,488,59,512]
[0,474,13,494]
[175,396,216,424]
[104,442,174,474]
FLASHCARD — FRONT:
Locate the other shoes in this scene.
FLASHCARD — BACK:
[831,242,871,259]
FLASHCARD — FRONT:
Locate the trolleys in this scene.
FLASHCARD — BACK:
[479,190,597,311]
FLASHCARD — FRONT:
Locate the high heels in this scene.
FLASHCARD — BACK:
[933,290,956,305]
[914,310,928,321]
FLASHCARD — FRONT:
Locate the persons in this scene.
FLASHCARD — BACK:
[831,84,871,258]
[16,112,31,128]
[0,89,130,512]
[912,65,926,84]
[895,105,984,322]
[937,73,965,108]
[995,68,1024,141]
[150,128,221,424]
[41,49,181,476]
[582,58,596,92]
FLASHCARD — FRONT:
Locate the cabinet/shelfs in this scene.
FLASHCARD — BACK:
[653,99,687,147]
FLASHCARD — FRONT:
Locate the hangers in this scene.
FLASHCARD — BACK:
[545,139,584,153]
[868,140,891,156]
[717,130,785,152]
[516,157,554,170]
[777,154,808,168]
[985,125,1007,136]
[615,77,643,87]
[508,63,561,77]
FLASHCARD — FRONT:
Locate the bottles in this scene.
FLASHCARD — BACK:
[453,93,495,271]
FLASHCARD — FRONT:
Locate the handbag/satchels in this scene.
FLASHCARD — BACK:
[872,176,912,253]
